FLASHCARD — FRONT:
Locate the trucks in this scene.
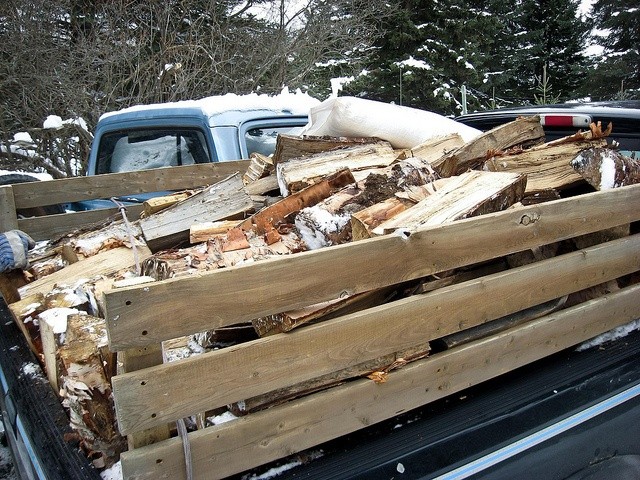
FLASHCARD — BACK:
[0,102,639,480]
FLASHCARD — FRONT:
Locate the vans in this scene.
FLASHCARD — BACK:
[72,97,313,212]
